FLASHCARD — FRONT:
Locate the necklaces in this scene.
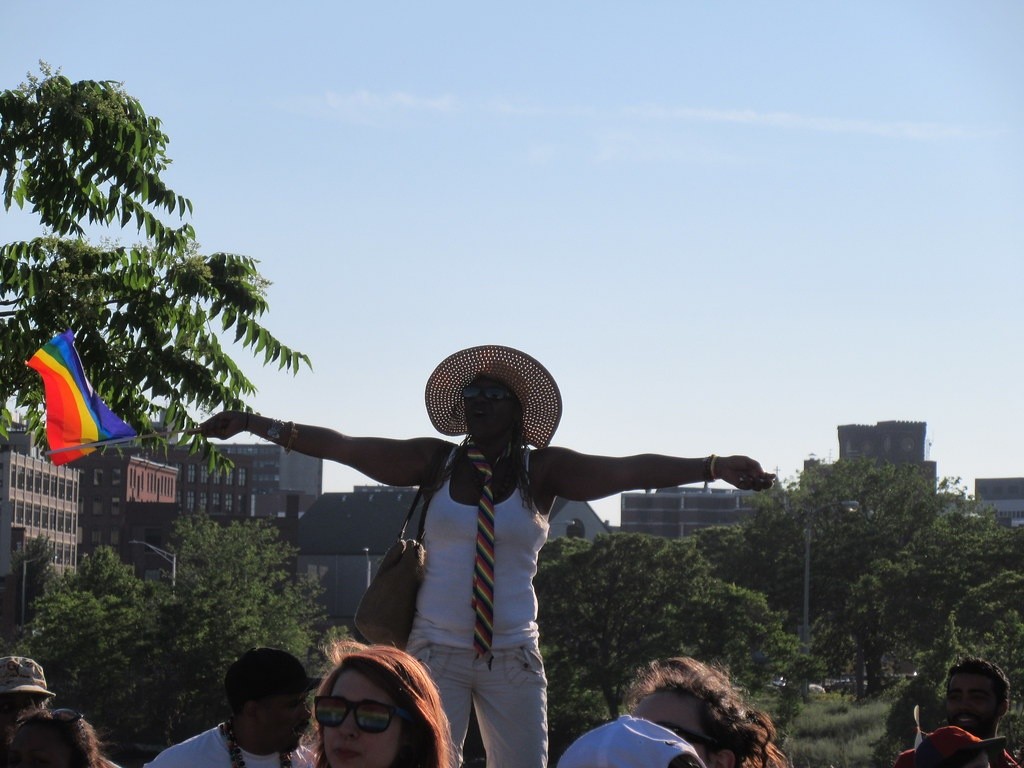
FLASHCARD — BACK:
[219,718,293,768]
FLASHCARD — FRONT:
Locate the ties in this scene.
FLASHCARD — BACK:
[466,451,509,655]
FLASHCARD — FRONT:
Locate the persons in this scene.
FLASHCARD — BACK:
[187,344,776,768]
[0,645,454,767]
[894,658,1022,768]
[559,658,794,768]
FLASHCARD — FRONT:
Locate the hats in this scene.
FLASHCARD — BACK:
[913,726,1008,768]
[224,647,323,706]
[894,748,916,768]
[556,714,705,768]
[425,344,563,449]
[0,656,56,699]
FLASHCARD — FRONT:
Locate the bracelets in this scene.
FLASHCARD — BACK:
[285,422,298,452]
[268,420,284,441]
[706,455,717,482]
[245,413,248,431]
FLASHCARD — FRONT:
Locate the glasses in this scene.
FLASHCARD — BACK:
[653,721,722,751]
[313,695,414,734]
[15,708,82,725]
[460,386,519,409]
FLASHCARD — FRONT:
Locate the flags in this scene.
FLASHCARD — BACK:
[24,328,138,466]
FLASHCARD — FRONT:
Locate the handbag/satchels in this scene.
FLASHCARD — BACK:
[355,440,455,649]
